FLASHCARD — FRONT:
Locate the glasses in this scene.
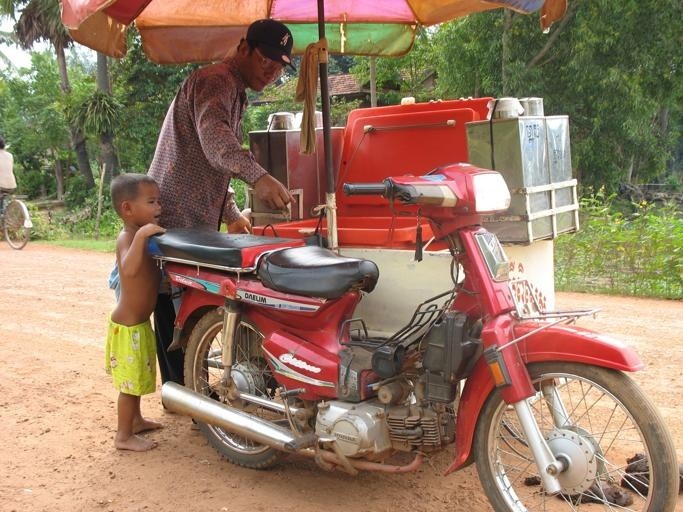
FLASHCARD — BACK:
[254,49,284,75]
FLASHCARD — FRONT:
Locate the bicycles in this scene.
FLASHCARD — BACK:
[0,192,32,250]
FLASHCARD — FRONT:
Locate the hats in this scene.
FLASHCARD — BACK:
[248,20,298,73]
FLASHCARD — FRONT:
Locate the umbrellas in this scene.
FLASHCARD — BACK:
[57,0,567,256]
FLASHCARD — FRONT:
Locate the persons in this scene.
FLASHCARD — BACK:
[145,19,297,389]
[104,173,167,452]
[0,136,18,199]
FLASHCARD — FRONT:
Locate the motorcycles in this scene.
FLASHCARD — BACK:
[147,160,679,512]
